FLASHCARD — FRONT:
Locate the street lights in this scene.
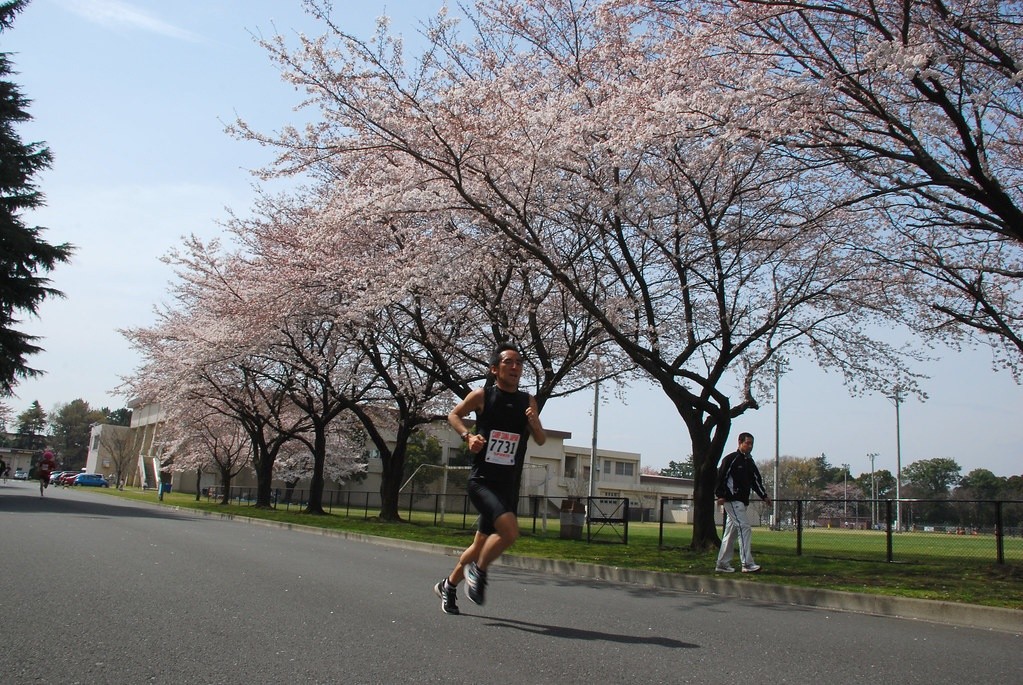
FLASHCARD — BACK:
[841,462,850,527]
[866,453,878,529]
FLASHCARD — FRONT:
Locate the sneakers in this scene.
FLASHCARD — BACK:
[463,561,486,605]
[715,564,735,572]
[742,564,761,572]
[434,577,459,615]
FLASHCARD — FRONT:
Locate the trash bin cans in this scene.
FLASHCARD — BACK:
[559,499,586,541]
[109,473,116,484]
[165,485,172,493]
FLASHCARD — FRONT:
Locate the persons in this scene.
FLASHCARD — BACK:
[433,343,546,615]
[3,463,11,484]
[0,455,6,477]
[714,433,772,573]
[38,451,56,496]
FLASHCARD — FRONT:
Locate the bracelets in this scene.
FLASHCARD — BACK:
[461,431,471,442]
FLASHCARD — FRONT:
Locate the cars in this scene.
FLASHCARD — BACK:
[13,471,27,480]
[49,471,109,488]
